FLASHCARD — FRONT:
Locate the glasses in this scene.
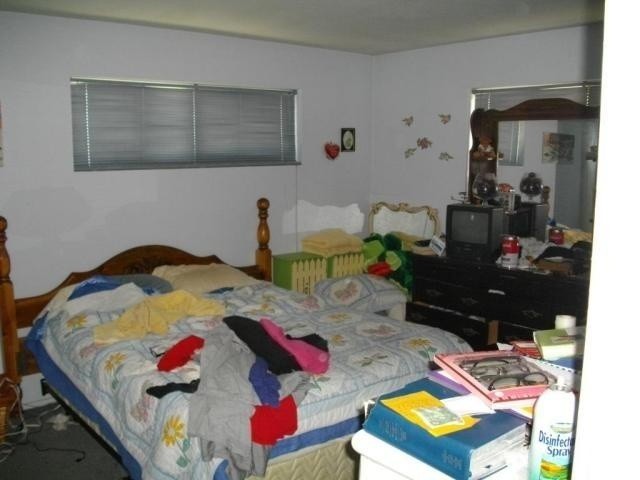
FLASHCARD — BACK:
[459,356,548,390]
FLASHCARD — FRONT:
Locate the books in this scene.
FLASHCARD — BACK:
[362,374,529,480]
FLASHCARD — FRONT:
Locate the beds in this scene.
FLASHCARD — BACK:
[0,198,476,479]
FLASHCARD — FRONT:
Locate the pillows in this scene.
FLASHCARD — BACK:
[151,261,263,296]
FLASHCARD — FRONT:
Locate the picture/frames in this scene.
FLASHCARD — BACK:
[340,128,356,153]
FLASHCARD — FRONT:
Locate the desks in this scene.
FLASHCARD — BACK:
[350,353,584,480]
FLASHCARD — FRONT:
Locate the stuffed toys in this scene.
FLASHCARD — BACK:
[472,135,497,160]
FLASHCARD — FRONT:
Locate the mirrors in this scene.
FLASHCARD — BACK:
[367,202,441,238]
[467,97,600,233]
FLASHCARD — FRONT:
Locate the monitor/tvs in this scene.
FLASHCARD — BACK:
[509,202,550,242]
[445,202,509,261]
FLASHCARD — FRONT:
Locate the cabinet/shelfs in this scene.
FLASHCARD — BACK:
[405,251,590,353]
